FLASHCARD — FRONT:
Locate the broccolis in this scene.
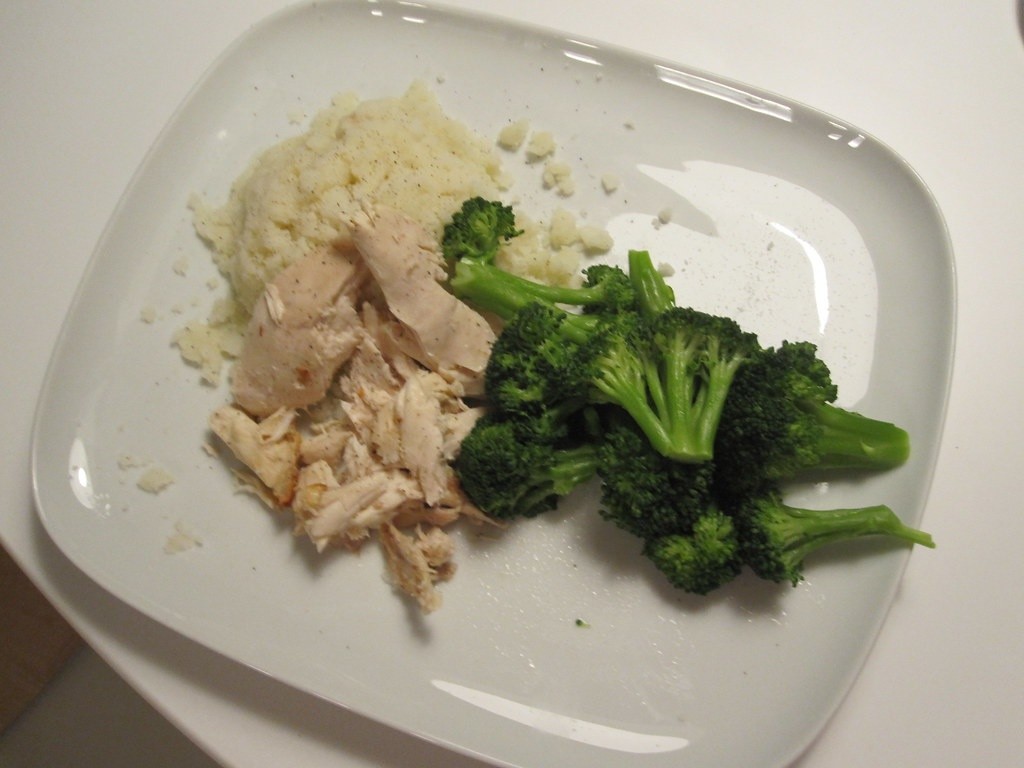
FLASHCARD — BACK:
[434,195,936,593]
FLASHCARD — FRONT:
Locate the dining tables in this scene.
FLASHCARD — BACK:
[0,1,1023,768]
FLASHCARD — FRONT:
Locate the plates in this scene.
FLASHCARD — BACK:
[31,0,957,768]
